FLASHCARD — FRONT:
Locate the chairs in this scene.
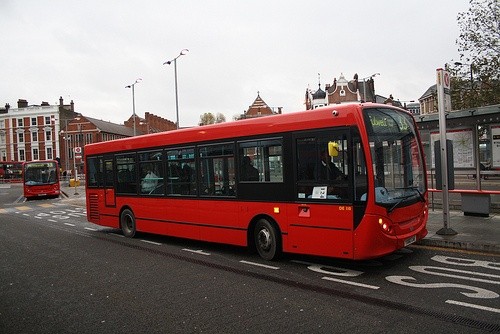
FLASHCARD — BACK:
[170,165,183,177]
[183,164,194,177]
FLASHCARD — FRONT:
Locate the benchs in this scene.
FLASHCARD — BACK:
[428,189,500,212]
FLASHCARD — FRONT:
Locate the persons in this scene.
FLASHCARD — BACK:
[244,156,259,180]
[132,162,193,194]
[314,151,343,182]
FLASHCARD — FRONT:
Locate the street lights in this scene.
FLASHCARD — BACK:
[139,122,150,134]
[124,78,143,136]
[454,62,488,107]
[51,117,81,187]
[351,72,381,102]
[163,49,190,129]
[63,136,71,181]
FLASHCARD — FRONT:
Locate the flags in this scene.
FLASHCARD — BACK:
[75,147,81,160]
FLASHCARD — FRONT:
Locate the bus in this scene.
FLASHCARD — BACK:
[83,100,429,262]
[21,159,61,199]
[0,160,25,183]
